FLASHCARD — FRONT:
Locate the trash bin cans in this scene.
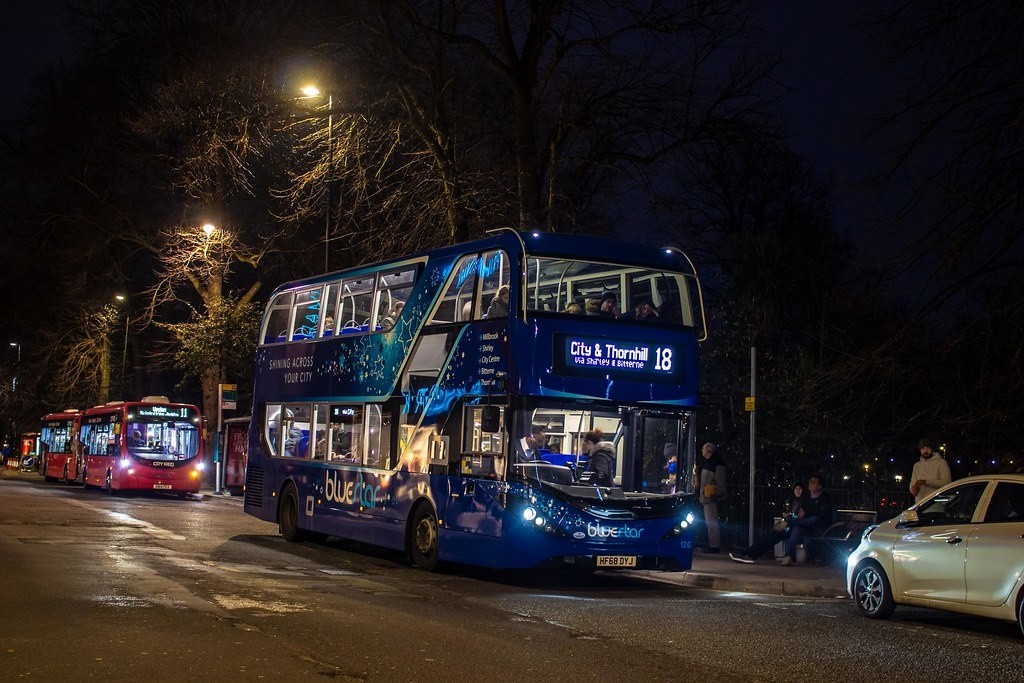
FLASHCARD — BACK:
[772,517,790,561]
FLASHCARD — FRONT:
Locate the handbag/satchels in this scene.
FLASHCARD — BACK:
[704,485,721,498]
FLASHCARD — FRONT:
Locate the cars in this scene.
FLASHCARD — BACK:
[846,475,1024,635]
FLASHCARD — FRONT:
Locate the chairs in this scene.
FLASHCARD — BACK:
[271,317,381,343]
[269,428,589,479]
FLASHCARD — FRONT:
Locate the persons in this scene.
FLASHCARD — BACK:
[729,473,835,565]
[910,439,952,515]
[332,422,349,456]
[381,301,404,330]
[128,430,145,446]
[488,285,509,318]
[621,300,661,319]
[560,302,586,314]
[169,446,177,456]
[325,316,334,330]
[514,426,545,479]
[305,429,326,460]
[28,441,33,454]
[583,426,616,487]
[544,435,560,454]
[585,291,621,319]
[699,443,726,553]
[662,442,703,553]
[270,407,304,457]
[154,441,164,451]
[148,441,154,448]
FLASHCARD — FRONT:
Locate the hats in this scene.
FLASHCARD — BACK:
[919,438,933,449]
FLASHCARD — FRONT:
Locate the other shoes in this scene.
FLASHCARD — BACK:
[775,520,787,531]
[703,547,720,553]
[780,555,793,565]
[728,549,755,564]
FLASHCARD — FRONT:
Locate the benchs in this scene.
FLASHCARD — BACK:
[783,509,878,567]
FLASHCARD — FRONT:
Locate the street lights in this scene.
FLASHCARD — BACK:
[203,222,223,386]
[116,295,130,399]
[300,82,333,273]
[10,342,21,374]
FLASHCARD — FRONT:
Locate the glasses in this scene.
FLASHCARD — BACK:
[808,480,821,485]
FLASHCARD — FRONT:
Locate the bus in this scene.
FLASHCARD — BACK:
[77,395,206,498]
[243,227,708,581]
[38,409,82,485]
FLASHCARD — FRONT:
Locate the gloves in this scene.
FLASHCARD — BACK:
[709,495,720,503]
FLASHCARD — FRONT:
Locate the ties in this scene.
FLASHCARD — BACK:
[526,450,531,459]
